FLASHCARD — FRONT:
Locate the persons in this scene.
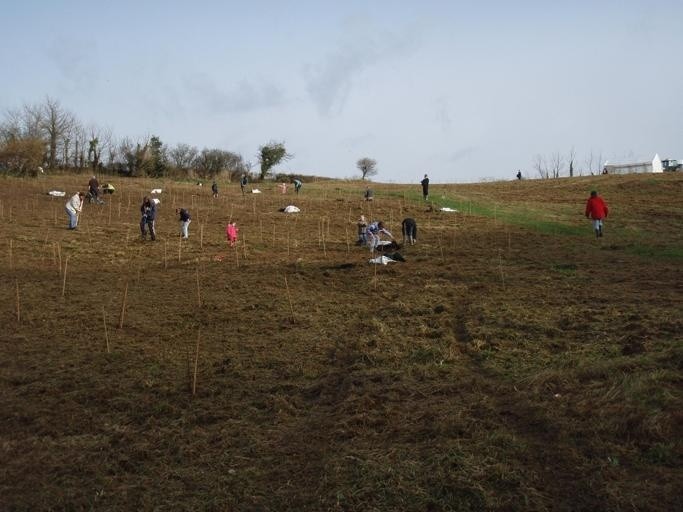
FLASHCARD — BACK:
[239,174,247,194]
[211,181,218,198]
[289,178,301,195]
[357,215,367,245]
[278,182,289,196]
[401,218,416,245]
[226,221,238,247]
[367,220,395,254]
[87,175,99,203]
[363,184,373,201]
[515,170,521,180]
[585,191,608,237]
[64,192,85,228]
[420,174,429,201]
[175,207,191,239]
[100,183,114,194]
[140,196,156,241]
